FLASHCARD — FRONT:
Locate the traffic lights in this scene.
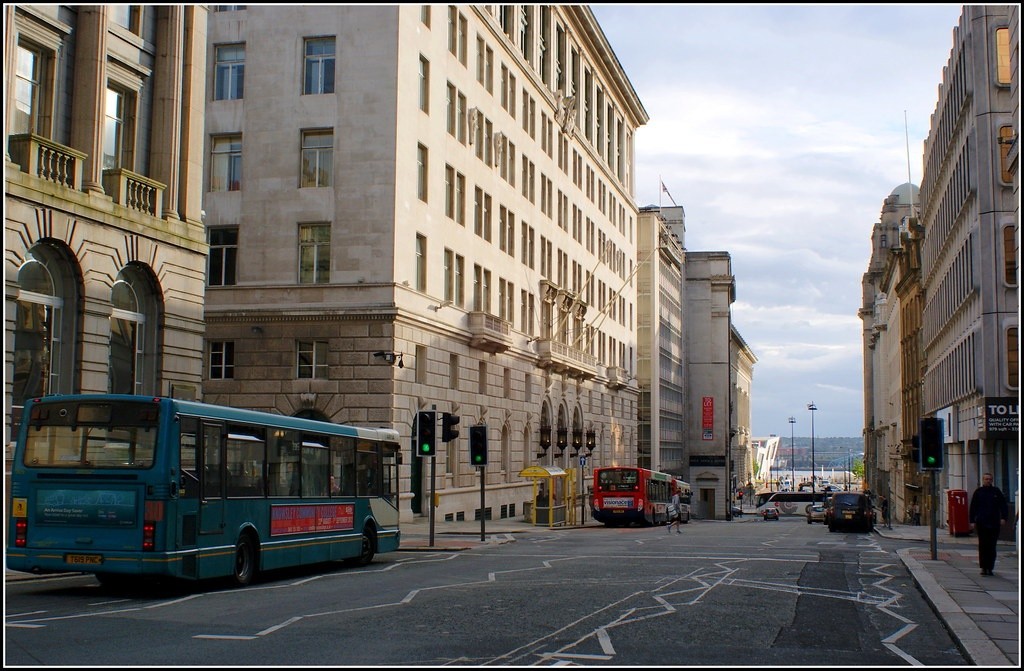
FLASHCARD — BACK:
[442,412,460,443]
[911,434,920,463]
[739,492,743,499]
[919,417,944,470]
[416,410,437,456]
[467,425,491,466]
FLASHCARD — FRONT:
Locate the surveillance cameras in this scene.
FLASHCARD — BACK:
[373,351,385,356]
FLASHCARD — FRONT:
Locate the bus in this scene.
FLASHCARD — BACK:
[671,479,696,524]
[590,466,674,526]
[5,394,403,590]
[758,491,828,514]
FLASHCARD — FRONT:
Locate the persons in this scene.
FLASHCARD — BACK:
[331,476,340,494]
[881,495,888,527]
[907,500,921,526]
[969,473,1009,576]
[666,490,682,534]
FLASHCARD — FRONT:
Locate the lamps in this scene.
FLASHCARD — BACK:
[526,335,540,344]
[681,247,688,251]
[677,240,683,245]
[672,234,679,238]
[435,300,453,311]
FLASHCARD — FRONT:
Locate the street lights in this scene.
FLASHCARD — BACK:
[808,402,818,506]
[788,416,799,493]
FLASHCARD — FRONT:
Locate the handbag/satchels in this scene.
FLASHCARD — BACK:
[669,505,678,518]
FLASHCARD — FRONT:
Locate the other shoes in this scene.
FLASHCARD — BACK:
[677,529,681,533]
[981,569,987,575]
[667,525,671,533]
[986,569,994,576]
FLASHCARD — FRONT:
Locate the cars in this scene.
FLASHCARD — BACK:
[777,477,843,493]
[732,507,743,518]
[763,508,779,521]
[755,492,775,508]
[807,492,877,531]
